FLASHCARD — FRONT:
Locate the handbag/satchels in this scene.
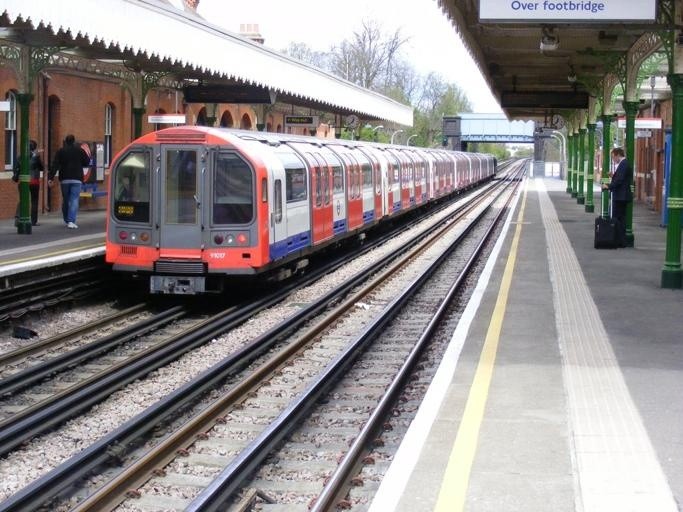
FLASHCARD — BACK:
[13,161,21,182]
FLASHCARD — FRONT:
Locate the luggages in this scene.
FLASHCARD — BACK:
[594,188,622,248]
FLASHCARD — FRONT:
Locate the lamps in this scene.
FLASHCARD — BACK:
[568,64,577,82]
[540,24,559,51]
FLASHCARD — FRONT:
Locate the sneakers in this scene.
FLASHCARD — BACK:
[68,222,78,228]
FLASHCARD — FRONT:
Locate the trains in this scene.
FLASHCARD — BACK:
[102,126,497,298]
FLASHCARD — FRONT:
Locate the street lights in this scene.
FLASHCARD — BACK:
[550,135,562,178]
[369,126,382,142]
[391,130,402,145]
[552,130,566,180]
[406,133,416,146]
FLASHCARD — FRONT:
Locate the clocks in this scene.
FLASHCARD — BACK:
[552,114,565,129]
[347,115,359,128]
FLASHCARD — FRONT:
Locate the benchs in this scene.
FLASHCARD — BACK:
[79,184,107,200]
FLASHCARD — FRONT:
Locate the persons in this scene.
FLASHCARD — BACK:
[47,134,90,229]
[12,140,44,226]
[603,148,633,248]
